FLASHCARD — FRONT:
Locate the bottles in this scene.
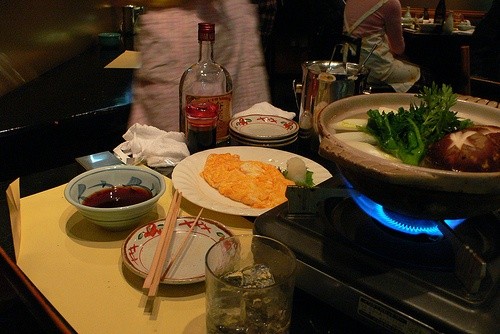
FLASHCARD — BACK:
[434,0,446,24]
[403,6,411,18]
[447,11,453,31]
[178,22,233,156]
[423,7,429,20]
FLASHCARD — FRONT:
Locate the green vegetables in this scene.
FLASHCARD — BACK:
[355,82,474,166]
[276,164,315,188]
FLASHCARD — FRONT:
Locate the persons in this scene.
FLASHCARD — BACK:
[471,0,500,103]
[344,0,440,95]
[112,0,270,145]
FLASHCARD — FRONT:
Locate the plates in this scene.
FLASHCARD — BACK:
[120,216,235,284]
[171,146,333,216]
[229,114,299,148]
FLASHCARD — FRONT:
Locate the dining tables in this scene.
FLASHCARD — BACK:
[403,26,474,94]
[0,116,337,334]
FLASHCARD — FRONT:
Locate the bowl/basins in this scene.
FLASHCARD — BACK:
[63,165,166,231]
[456,25,471,31]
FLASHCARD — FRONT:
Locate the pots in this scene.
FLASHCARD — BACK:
[317,93,500,218]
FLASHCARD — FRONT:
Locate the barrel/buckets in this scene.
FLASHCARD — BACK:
[293,60,372,141]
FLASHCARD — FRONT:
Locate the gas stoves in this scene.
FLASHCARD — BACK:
[252,172,500,334]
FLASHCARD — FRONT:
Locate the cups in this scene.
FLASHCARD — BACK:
[205,234,296,334]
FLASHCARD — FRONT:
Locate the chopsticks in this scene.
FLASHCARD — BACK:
[142,189,182,297]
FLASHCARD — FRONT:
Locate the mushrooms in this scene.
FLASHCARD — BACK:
[426,125,500,174]
